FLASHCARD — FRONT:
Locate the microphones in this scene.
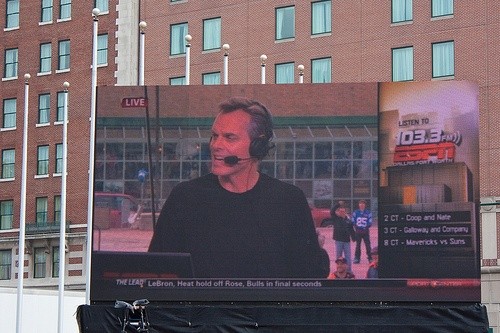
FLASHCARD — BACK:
[223,151,265,165]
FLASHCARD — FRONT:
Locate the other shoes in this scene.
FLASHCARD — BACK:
[354,259,360,263]
[368,259,373,264]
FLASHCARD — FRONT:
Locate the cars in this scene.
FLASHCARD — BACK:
[309,203,335,227]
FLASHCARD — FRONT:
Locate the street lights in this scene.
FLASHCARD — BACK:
[139,22,149,86]
[222,43,231,85]
[259,54,269,85]
[185,34,193,84]
[298,64,306,84]
[89,7,102,306]
[58,81,70,333]
[16,73,31,332]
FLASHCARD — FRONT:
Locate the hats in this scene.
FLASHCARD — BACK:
[335,257,347,263]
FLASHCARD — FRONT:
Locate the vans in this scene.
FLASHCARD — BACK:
[93,192,148,229]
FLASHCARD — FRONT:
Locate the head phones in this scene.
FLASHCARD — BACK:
[249,125,273,157]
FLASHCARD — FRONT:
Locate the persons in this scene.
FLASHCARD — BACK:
[144,98,377,280]
